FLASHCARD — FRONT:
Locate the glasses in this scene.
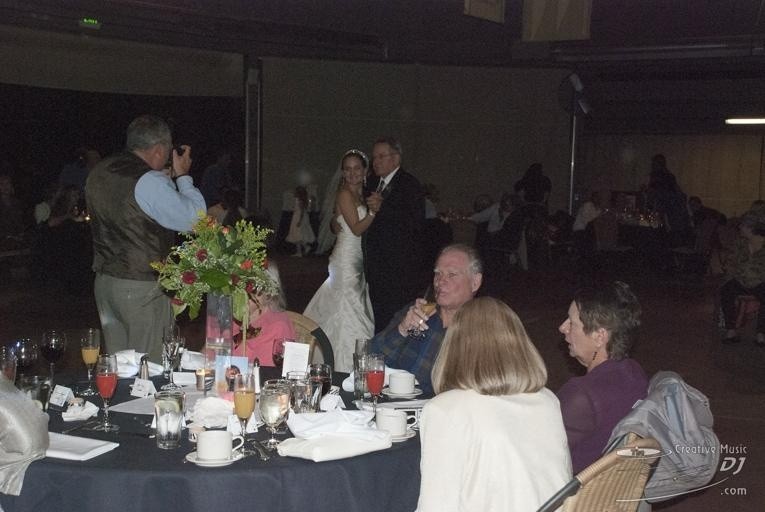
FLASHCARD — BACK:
[372,152,396,160]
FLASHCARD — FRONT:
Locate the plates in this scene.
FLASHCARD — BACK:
[185,452,244,467]
[392,430,416,444]
[382,388,423,399]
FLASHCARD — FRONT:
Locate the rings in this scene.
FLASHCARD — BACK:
[422,311,426,318]
[419,319,423,326]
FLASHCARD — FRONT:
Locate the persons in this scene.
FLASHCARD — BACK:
[424,183,439,219]
[648,155,679,238]
[719,212,765,348]
[0,176,26,235]
[514,162,552,265]
[66,133,101,172]
[206,186,232,223]
[33,181,71,237]
[198,148,239,206]
[363,243,486,392]
[473,194,491,211]
[751,200,765,216]
[206,263,295,367]
[577,191,605,246]
[689,196,705,223]
[302,148,383,372]
[496,194,526,268]
[85,116,207,365]
[285,186,315,258]
[417,297,573,512]
[0,371,49,496]
[61,186,81,214]
[221,190,242,226]
[556,281,651,476]
[329,137,425,334]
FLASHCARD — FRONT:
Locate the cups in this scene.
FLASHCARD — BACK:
[375,409,417,437]
[295,379,323,414]
[40,330,66,390]
[286,372,308,409]
[17,338,40,388]
[154,391,185,451]
[196,430,244,460]
[388,372,415,393]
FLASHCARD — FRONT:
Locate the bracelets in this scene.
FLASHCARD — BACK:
[368,211,376,218]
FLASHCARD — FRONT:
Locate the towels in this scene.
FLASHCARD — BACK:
[277,429,392,462]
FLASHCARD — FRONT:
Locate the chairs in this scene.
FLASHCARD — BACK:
[286,310,335,372]
[536,439,662,512]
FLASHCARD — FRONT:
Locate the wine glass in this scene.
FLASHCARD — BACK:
[264,379,292,435]
[162,324,181,392]
[234,374,256,442]
[92,353,120,432]
[365,353,386,427]
[407,285,441,340]
[306,363,332,412]
[260,385,289,449]
[272,337,295,378]
[354,353,373,412]
[78,328,101,396]
[354,339,373,399]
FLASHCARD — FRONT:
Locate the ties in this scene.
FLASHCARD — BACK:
[377,180,385,194]
